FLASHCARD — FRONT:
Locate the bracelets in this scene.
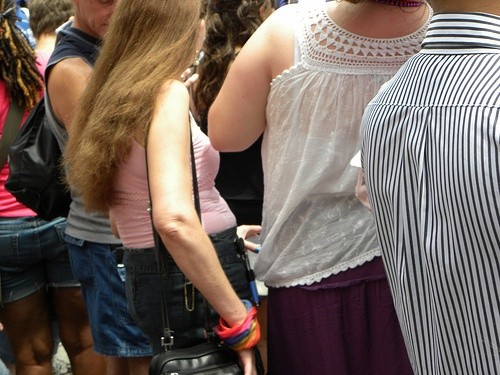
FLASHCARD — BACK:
[214,300,261,350]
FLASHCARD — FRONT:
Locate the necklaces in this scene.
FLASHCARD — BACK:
[372,0,425,6]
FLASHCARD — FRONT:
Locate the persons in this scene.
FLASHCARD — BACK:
[26,0,75,57]
[207,0,434,375]
[66,0,263,375]
[360,0,500,375]
[0,0,106,375]
[44,0,161,375]
[188,0,302,375]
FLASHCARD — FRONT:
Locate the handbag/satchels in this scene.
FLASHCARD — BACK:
[6,96,72,217]
[148,342,242,375]
